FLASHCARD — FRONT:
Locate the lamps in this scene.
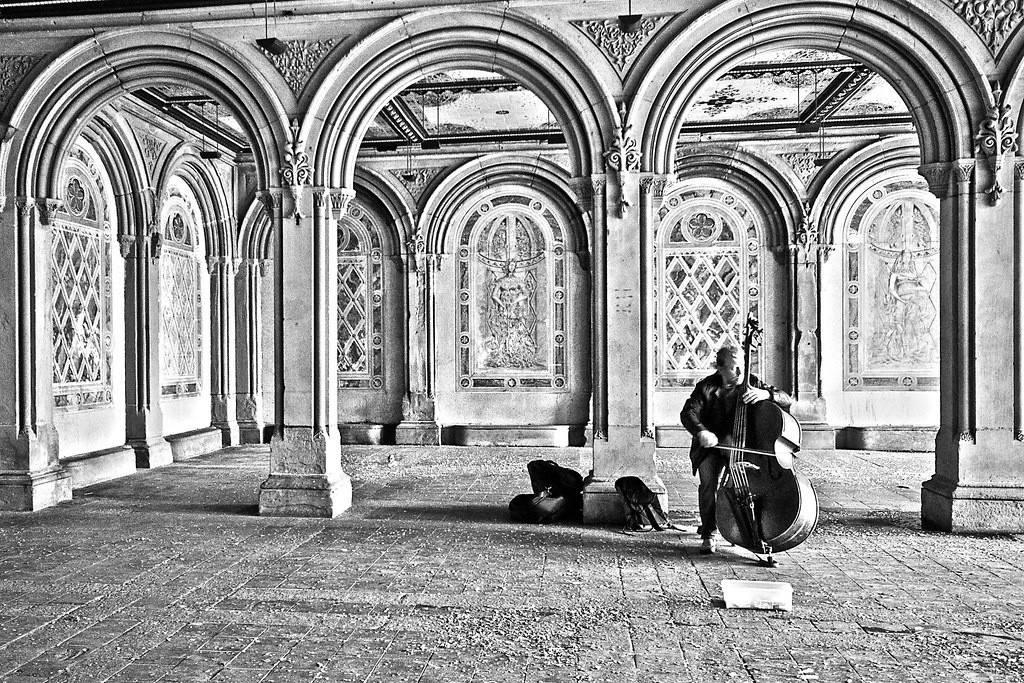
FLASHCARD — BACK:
[256,0,286,55]
[420,89,443,150]
[199,102,223,159]
[796,72,820,134]
[618,0,642,33]
[814,116,829,165]
[401,143,417,182]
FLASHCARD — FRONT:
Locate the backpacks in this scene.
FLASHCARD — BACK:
[615,476,673,532]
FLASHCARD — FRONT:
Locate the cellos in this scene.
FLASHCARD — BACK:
[715,312,819,554]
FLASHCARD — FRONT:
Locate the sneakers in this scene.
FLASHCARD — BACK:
[700,533,719,553]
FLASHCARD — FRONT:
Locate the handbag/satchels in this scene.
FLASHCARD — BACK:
[527,460,583,504]
[508,489,566,524]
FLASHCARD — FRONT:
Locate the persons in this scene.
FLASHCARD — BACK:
[680,346,793,554]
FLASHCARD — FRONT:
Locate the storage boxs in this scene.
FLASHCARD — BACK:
[721,577,795,611]
